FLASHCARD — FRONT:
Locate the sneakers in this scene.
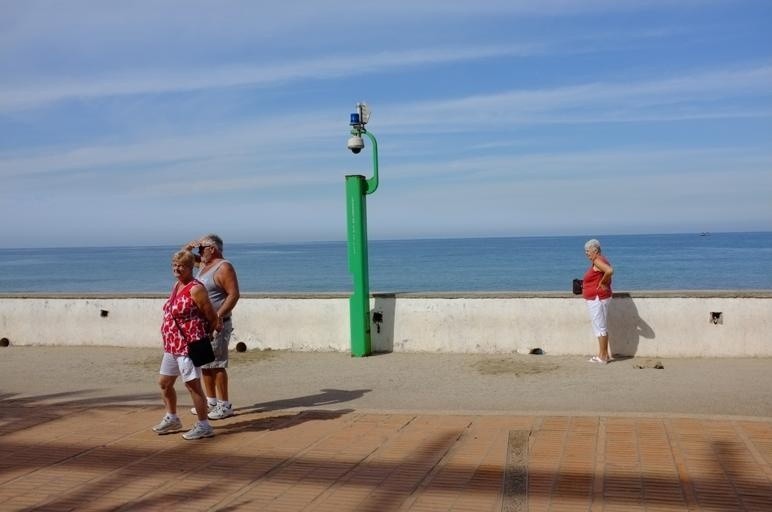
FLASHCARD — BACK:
[182,422,214,439]
[152,416,182,433]
[191,402,234,420]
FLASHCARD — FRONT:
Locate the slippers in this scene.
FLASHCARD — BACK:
[589,354,609,364]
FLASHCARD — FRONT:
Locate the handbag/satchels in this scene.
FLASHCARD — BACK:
[573,278,583,295]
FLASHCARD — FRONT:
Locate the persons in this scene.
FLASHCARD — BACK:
[152,234,240,440]
[581,239,613,365]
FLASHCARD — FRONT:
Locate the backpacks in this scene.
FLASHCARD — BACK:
[189,338,215,367]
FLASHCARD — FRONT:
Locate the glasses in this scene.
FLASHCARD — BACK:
[199,246,210,250]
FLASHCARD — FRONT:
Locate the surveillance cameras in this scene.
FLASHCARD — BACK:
[348,136,364,154]
